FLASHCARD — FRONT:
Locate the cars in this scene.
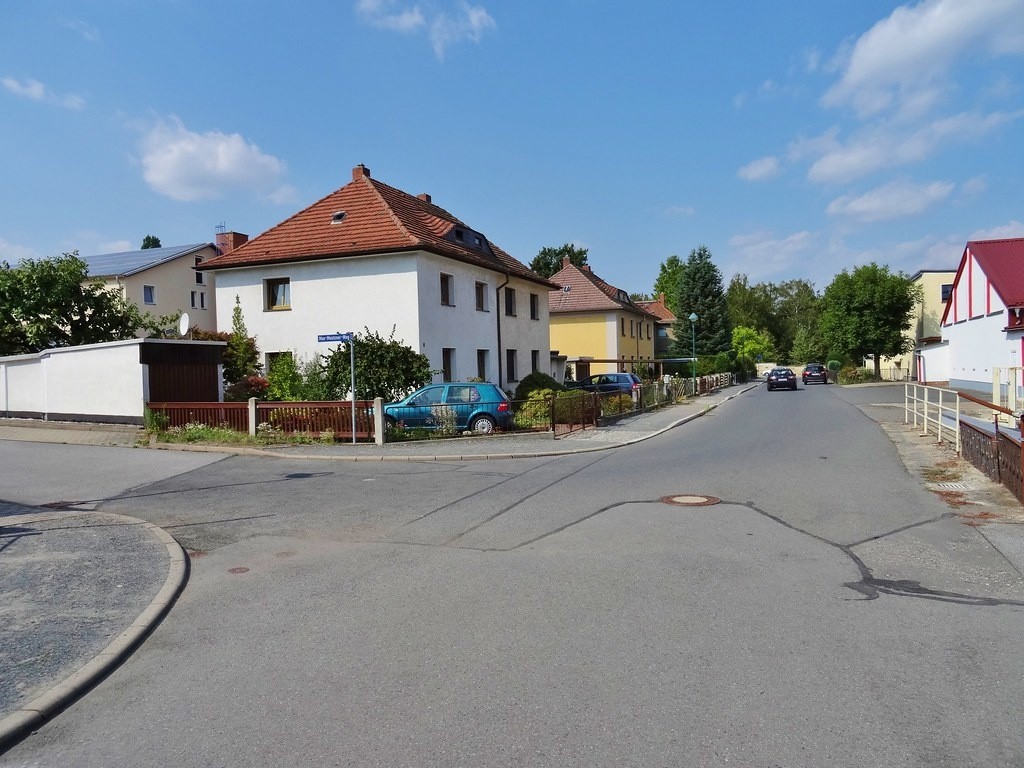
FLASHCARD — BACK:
[767,368,797,391]
[565,373,641,394]
[802,364,828,384]
[762,367,787,376]
[365,379,514,436]
[662,358,698,366]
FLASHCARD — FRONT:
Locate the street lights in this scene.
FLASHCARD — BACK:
[688,312,699,397]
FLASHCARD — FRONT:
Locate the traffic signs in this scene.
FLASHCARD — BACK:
[318,335,350,341]
